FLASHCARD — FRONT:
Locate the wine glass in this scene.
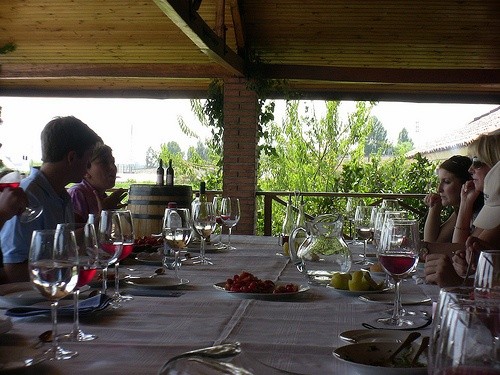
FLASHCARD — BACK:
[29,197,242,365]
[354,204,420,323]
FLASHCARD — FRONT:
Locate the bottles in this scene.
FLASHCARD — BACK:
[283,200,294,246]
[296,201,307,228]
[156,159,164,185]
[166,159,175,185]
[198,181,208,202]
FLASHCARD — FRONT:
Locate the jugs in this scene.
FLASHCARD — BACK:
[289,212,352,285]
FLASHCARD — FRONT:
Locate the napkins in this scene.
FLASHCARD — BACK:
[8,293,113,317]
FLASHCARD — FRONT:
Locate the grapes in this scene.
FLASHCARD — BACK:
[225,271,257,293]
[134,235,163,244]
[275,284,295,293]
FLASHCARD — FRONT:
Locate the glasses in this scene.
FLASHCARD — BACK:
[472,155,485,169]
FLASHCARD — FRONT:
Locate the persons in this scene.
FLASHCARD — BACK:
[0,115,129,285]
[399,130,500,294]
[66,145,117,228]
[0,187,30,284]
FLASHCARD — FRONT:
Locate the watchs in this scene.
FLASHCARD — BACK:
[419,241,429,262]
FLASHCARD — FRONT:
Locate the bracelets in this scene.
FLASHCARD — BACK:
[454,226,470,230]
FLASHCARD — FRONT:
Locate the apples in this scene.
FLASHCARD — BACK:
[332,270,373,291]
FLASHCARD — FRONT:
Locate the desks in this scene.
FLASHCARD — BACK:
[0,233,500,375]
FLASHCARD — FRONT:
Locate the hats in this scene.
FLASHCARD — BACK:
[472,161,500,230]
[91,145,112,162]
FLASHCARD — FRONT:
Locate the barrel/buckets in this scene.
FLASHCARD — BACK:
[127,185,195,242]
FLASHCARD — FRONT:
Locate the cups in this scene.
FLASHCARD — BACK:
[429,250,500,375]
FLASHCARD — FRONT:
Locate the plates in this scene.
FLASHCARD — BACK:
[124,276,190,288]
[212,280,311,297]
[91,272,132,284]
[326,282,395,295]
[338,330,428,345]
[359,292,432,304]
[357,252,378,263]
[360,265,416,276]
[183,243,230,250]
[331,343,428,371]
[10,292,89,307]
[135,252,189,264]
